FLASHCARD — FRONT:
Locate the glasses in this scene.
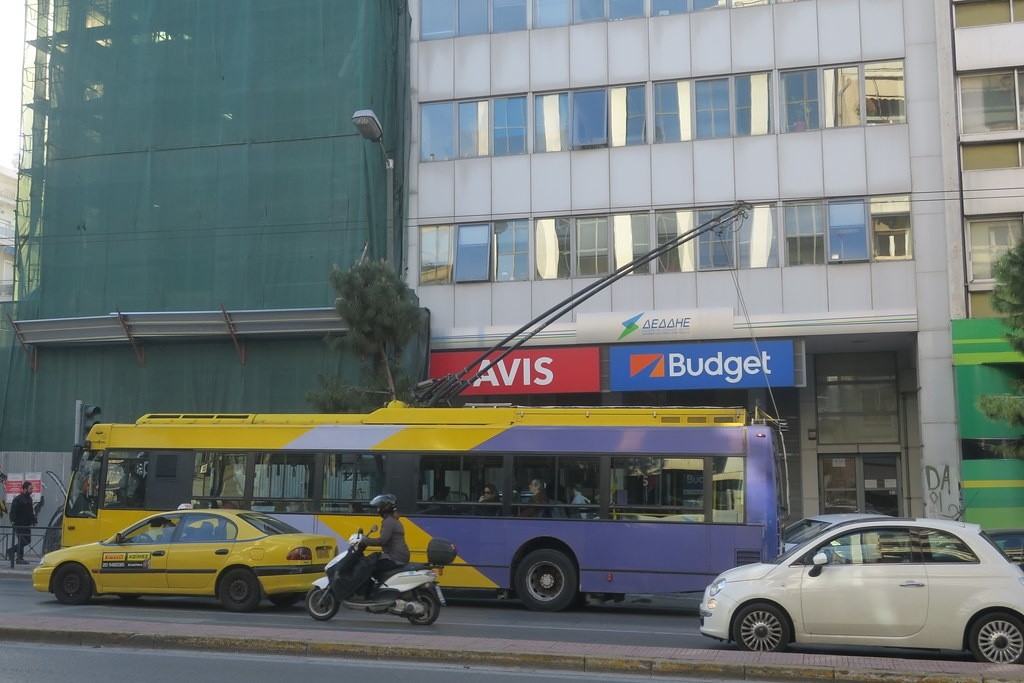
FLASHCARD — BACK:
[481,491,490,496]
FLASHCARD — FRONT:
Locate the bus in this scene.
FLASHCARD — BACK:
[60,201,778,613]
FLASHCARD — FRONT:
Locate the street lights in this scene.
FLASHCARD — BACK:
[351,110,394,277]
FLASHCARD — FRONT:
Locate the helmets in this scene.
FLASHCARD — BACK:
[369,494,397,515]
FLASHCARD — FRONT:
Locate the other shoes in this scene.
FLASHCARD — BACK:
[7,549,12,560]
[16,559,29,564]
[349,592,365,602]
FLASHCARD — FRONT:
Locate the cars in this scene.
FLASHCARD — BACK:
[699,509,1024,664]
[33,504,338,613]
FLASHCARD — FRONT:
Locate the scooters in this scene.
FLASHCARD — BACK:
[305,524,457,626]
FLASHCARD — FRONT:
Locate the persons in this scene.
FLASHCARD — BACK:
[106,460,589,519]
[159,522,175,541]
[6,481,36,564]
[349,494,411,600]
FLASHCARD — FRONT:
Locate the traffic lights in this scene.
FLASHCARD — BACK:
[81,405,102,440]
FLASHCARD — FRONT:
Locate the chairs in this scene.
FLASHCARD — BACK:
[214,526,227,540]
